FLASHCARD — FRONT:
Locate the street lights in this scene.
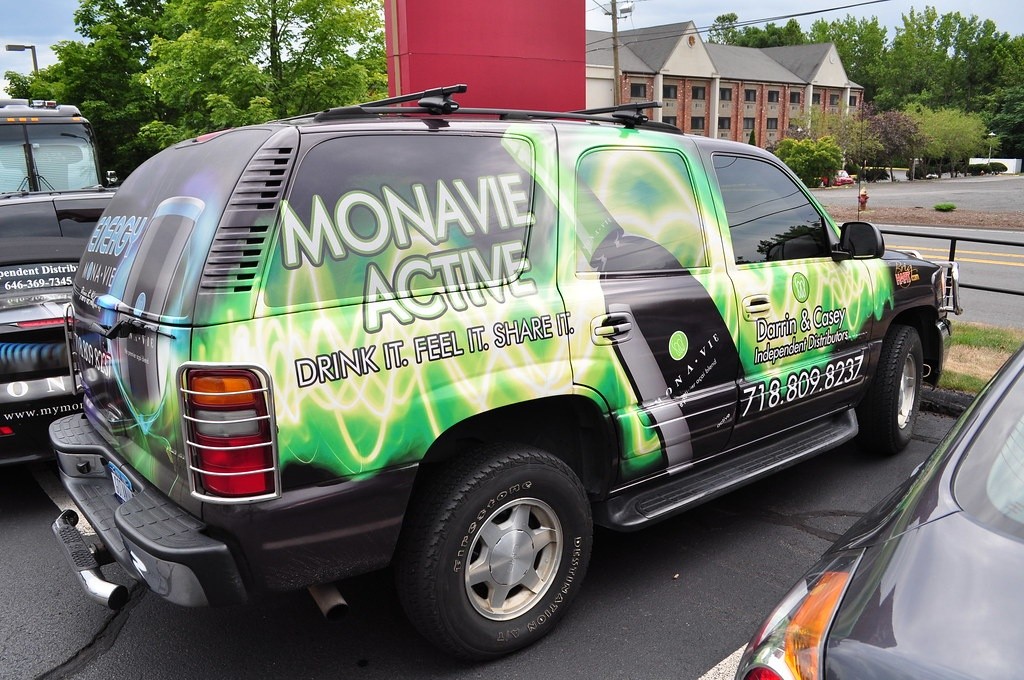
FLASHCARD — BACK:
[988,131,996,165]
[6,45,38,73]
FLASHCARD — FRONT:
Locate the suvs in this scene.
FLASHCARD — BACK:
[50,85,965,660]
[0,188,136,472]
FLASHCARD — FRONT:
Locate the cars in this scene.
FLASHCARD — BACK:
[734,339,1024,680]
[823,169,853,186]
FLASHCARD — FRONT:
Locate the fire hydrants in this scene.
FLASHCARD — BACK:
[857,186,868,209]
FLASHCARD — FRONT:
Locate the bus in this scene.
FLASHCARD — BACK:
[0,94,118,193]
